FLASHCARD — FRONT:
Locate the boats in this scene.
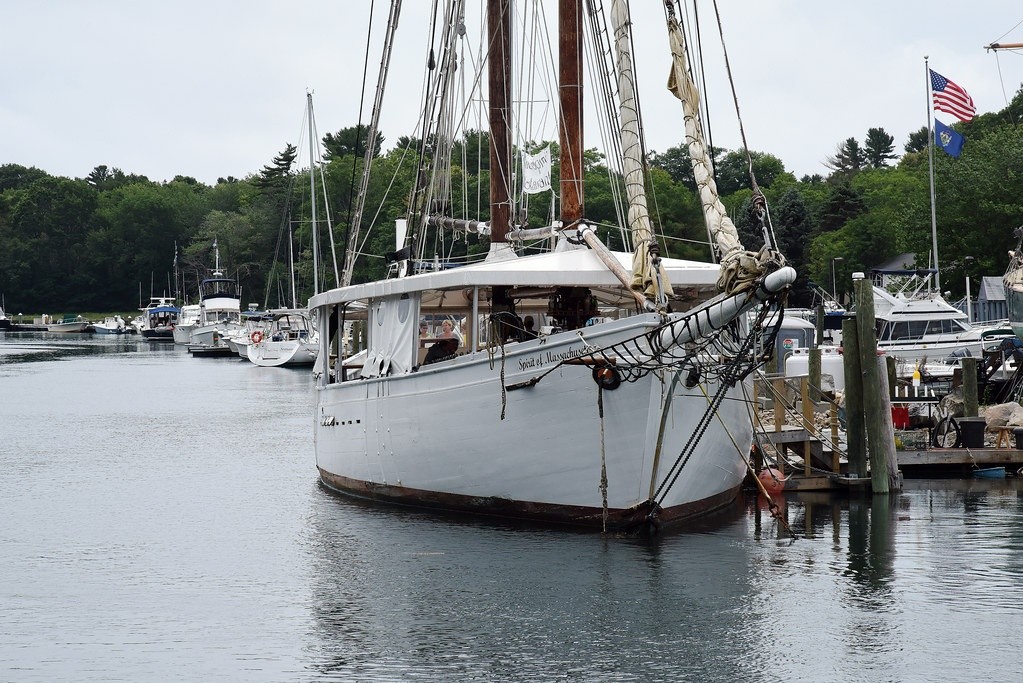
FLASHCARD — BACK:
[136,270,181,341]
[749,249,1023,401]
[221,311,262,359]
[246,90,338,367]
[49,315,88,333]
[14,313,53,331]
[173,287,201,345]
[92,315,132,334]
[0,293,13,328]
[188,235,243,357]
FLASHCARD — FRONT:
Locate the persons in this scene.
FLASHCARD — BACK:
[523,316,538,340]
[437,319,460,340]
[418,323,433,339]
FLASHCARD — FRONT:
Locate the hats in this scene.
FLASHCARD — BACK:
[523,316,533,325]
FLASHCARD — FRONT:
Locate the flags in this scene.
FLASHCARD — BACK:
[930,68,978,123]
[934,118,966,158]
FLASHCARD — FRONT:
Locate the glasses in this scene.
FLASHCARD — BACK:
[423,326,428,329]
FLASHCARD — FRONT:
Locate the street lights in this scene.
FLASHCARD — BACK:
[832,258,844,299]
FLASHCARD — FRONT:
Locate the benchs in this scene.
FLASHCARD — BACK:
[995,425,1018,449]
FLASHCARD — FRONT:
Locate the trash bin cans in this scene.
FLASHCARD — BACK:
[63,312,77,323]
[957,418,988,449]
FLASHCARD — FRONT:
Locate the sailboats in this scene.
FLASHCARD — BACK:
[310,0,800,533]
[985,19,1023,343]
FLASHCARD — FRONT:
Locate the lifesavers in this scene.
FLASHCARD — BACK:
[251,330,263,343]
